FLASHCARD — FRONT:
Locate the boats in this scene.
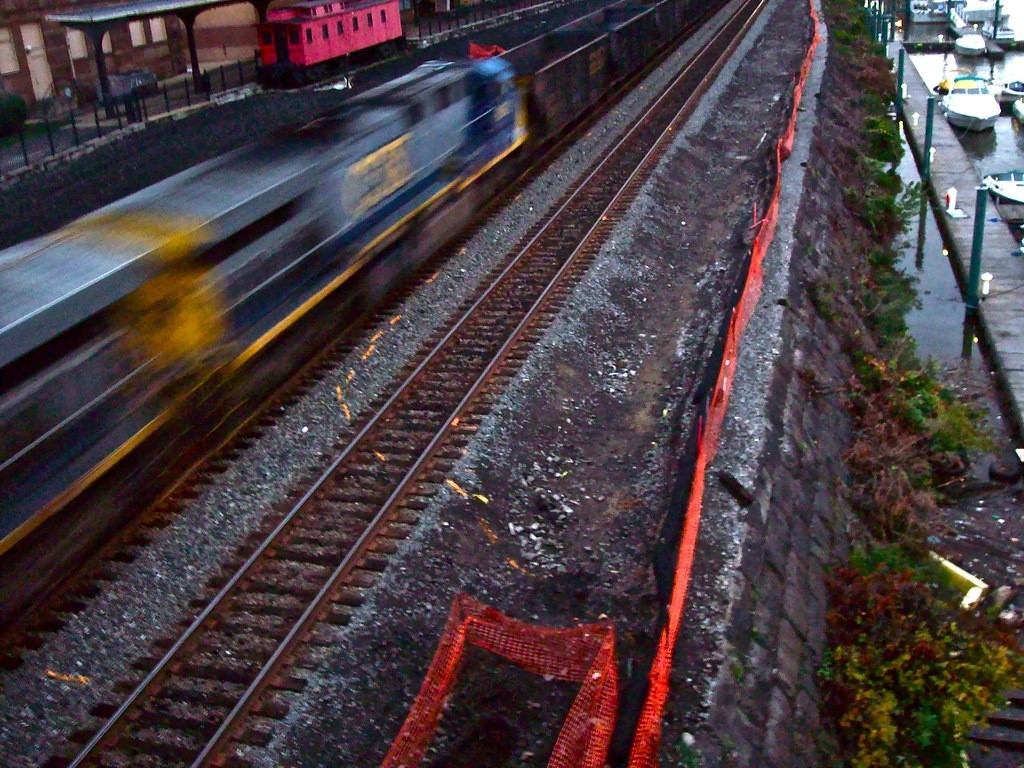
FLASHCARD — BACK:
[984,171,1024,224]
[911,0,1005,24]
[981,12,1015,48]
[938,72,1024,131]
[956,34,985,55]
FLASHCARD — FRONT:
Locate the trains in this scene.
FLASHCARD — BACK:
[0,0,722,570]
